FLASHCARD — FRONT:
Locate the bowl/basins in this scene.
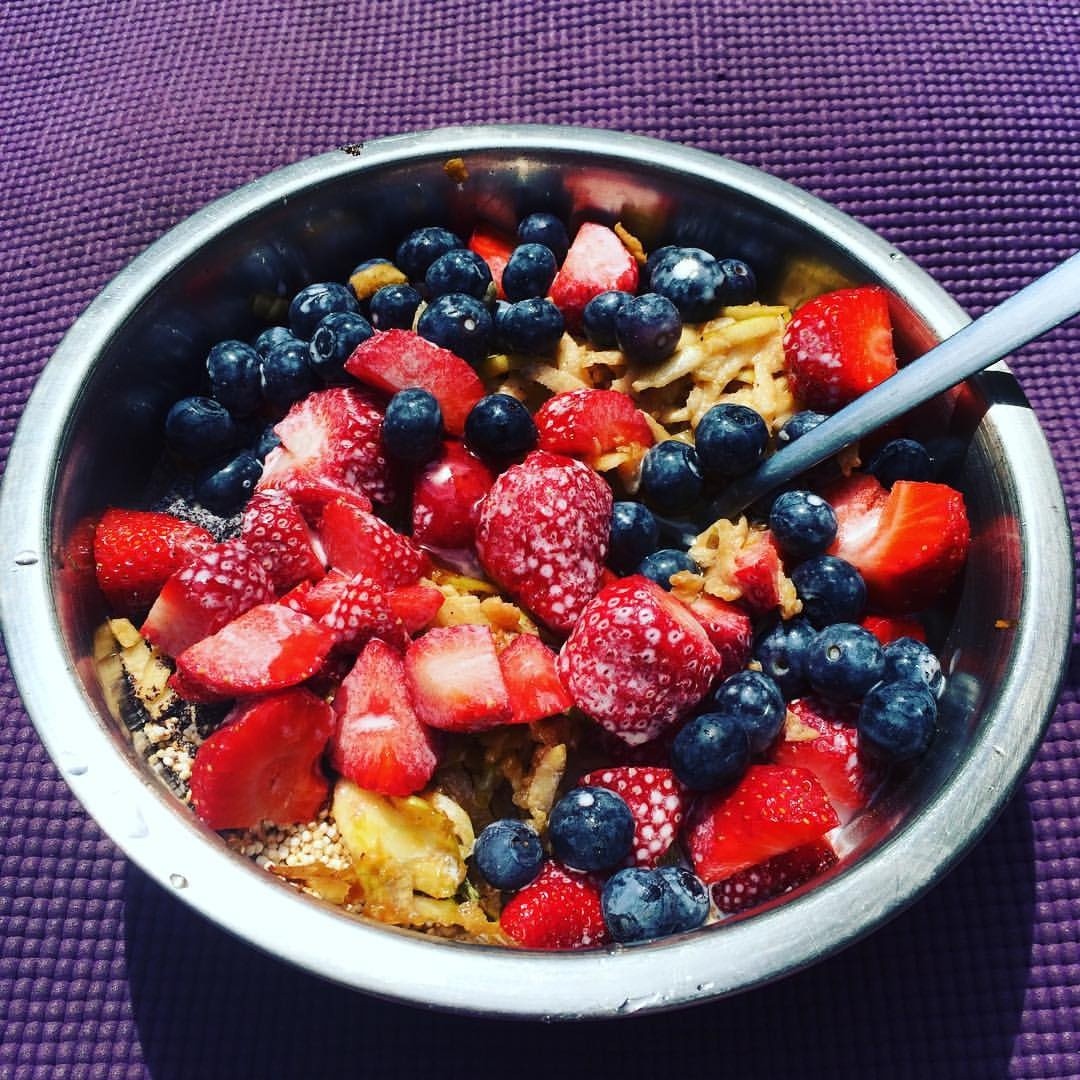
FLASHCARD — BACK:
[0,121,1074,1023]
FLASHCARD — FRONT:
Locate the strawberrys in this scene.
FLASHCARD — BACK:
[84,220,972,951]
